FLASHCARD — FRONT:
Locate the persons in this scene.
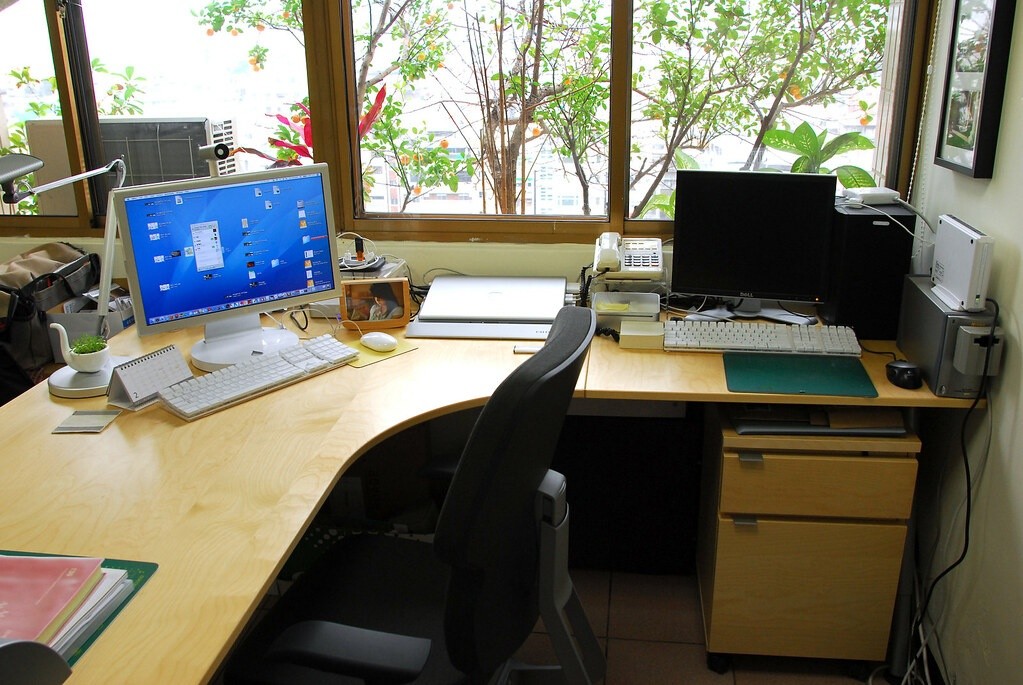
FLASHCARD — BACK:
[369,283,403,320]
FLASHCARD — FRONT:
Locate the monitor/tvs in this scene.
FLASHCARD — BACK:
[670,170,837,325]
[112,162,343,372]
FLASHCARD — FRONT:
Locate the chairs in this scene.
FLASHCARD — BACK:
[223,306,604,685]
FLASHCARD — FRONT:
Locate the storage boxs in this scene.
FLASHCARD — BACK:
[593,292,660,330]
[45,276,136,365]
[619,320,664,351]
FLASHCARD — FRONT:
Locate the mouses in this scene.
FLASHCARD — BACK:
[886,359,923,390]
[360,331,398,352]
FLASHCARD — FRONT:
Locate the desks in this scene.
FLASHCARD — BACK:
[0,297,989,685]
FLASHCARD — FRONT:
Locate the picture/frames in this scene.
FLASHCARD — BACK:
[934,0,1017,181]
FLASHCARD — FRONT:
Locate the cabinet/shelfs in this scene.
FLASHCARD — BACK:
[695,405,924,681]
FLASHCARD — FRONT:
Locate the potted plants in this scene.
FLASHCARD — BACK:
[48,322,110,373]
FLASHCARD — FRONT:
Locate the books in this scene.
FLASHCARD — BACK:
[0,556,134,662]
[87,282,129,312]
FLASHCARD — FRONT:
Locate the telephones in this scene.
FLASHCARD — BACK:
[593,232,665,282]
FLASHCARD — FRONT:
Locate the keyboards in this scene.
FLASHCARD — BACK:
[158,334,360,423]
[663,320,862,357]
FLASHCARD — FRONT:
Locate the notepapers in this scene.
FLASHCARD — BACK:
[618,321,665,349]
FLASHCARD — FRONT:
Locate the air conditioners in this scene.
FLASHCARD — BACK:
[25,114,235,216]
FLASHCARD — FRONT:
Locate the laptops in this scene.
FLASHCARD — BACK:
[418,275,567,325]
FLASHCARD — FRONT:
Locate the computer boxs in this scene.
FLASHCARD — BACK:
[815,194,917,340]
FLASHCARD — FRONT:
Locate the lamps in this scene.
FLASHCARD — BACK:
[0,152,127,338]
